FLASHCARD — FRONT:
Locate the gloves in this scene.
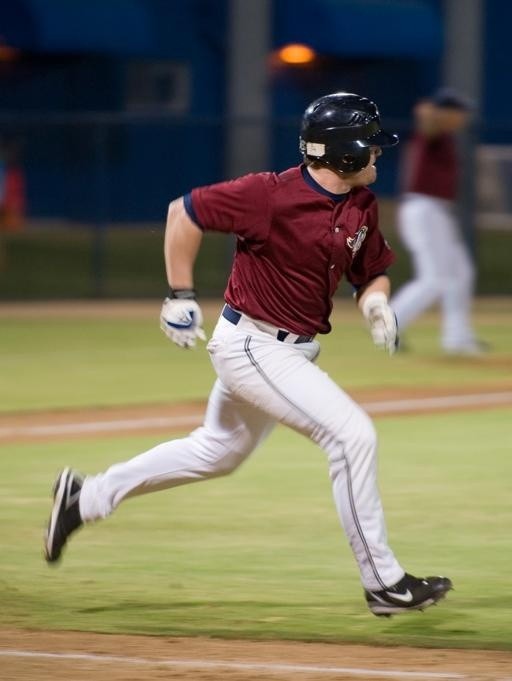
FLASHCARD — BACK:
[159,289,209,353]
[363,291,401,358]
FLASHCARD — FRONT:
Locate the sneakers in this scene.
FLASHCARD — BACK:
[365,571,453,619]
[42,466,84,563]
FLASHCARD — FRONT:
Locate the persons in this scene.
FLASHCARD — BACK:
[42,88,457,622]
[386,95,485,358]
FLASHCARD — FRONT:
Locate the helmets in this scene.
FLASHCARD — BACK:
[297,91,399,174]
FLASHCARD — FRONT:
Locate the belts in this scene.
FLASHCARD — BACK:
[221,305,314,345]
